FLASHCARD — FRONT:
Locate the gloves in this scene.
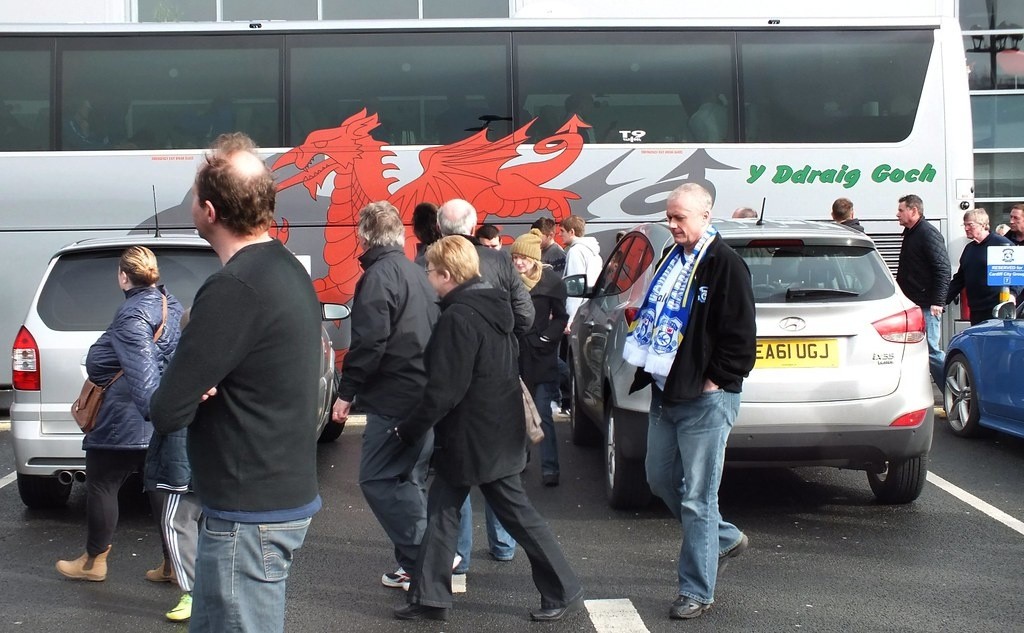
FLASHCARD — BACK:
[383,425,404,445]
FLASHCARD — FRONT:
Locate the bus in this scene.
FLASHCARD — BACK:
[0,18,973,386]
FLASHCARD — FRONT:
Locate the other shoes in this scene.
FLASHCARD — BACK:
[146,559,177,584]
[56,545,112,580]
[544,476,558,486]
[939,412,947,418]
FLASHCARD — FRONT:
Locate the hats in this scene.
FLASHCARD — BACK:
[511,228,542,260]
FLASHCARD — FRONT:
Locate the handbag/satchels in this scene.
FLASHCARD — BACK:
[72,378,104,434]
[519,376,544,444]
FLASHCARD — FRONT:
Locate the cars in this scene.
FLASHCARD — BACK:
[8,233,352,508]
[943,287,1024,441]
[561,219,935,509]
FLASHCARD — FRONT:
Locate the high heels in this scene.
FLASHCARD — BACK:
[531,595,584,621]
[394,605,448,620]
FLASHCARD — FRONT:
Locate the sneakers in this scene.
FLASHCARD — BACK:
[382,552,467,592]
[165,594,193,621]
[716,534,748,577]
[669,595,710,619]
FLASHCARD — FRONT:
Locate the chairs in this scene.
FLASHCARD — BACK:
[753,246,838,303]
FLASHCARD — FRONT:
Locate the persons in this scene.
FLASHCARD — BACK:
[141,130,321,633]
[55,247,185,582]
[331,181,1023,633]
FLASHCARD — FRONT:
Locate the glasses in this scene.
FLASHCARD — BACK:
[424,268,440,277]
